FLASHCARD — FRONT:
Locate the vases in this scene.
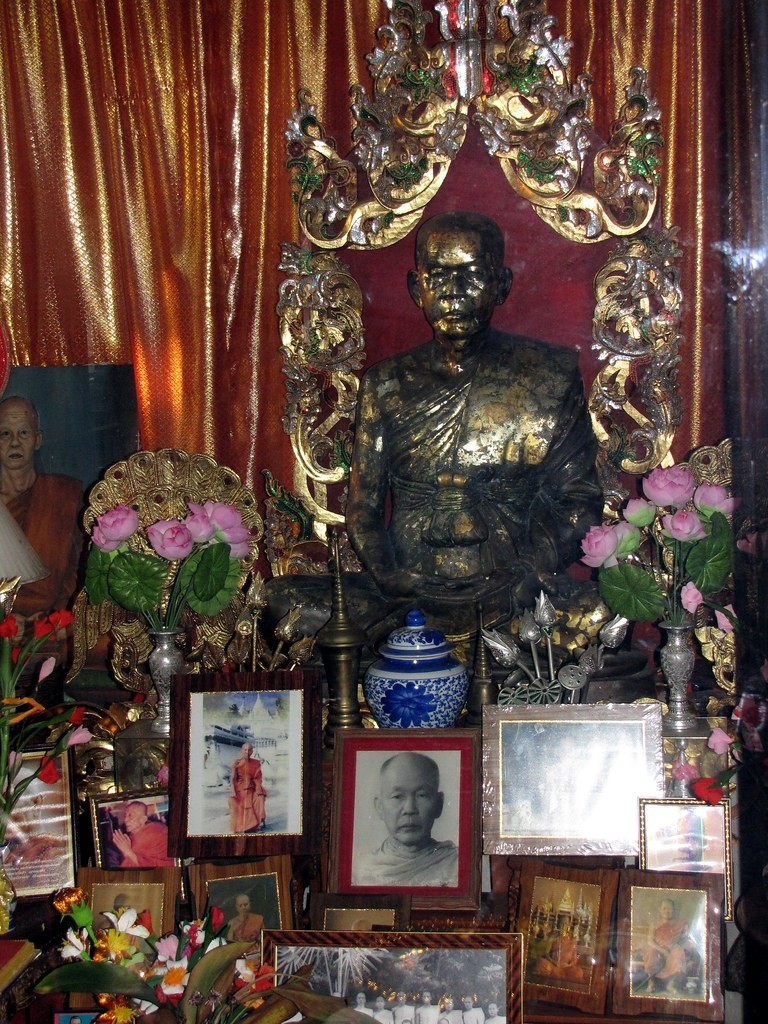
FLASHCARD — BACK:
[151,630,181,731]
[363,610,466,728]
[659,619,698,733]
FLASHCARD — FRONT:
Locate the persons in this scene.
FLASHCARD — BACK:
[351,920,371,931]
[111,802,172,868]
[258,212,604,650]
[532,911,583,982]
[99,894,156,936]
[70,1015,82,1024]
[229,742,268,834]
[642,897,698,984]
[329,992,506,1024]
[228,893,264,944]
[352,752,459,887]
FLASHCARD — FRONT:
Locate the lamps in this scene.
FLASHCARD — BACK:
[0,504,51,585]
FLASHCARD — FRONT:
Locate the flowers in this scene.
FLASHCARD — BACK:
[0,459,768,1024]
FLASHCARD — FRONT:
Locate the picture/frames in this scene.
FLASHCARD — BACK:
[516,858,618,1016]
[170,671,318,857]
[484,702,665,856]
[328,728,483,912]
[67,868,181,1010]
[3,744,82,905]
[611,869,724,1022]
[189,853,294,956]
[637,796,734,924]
[258,929,526,1024]
[90,788,187,907]
